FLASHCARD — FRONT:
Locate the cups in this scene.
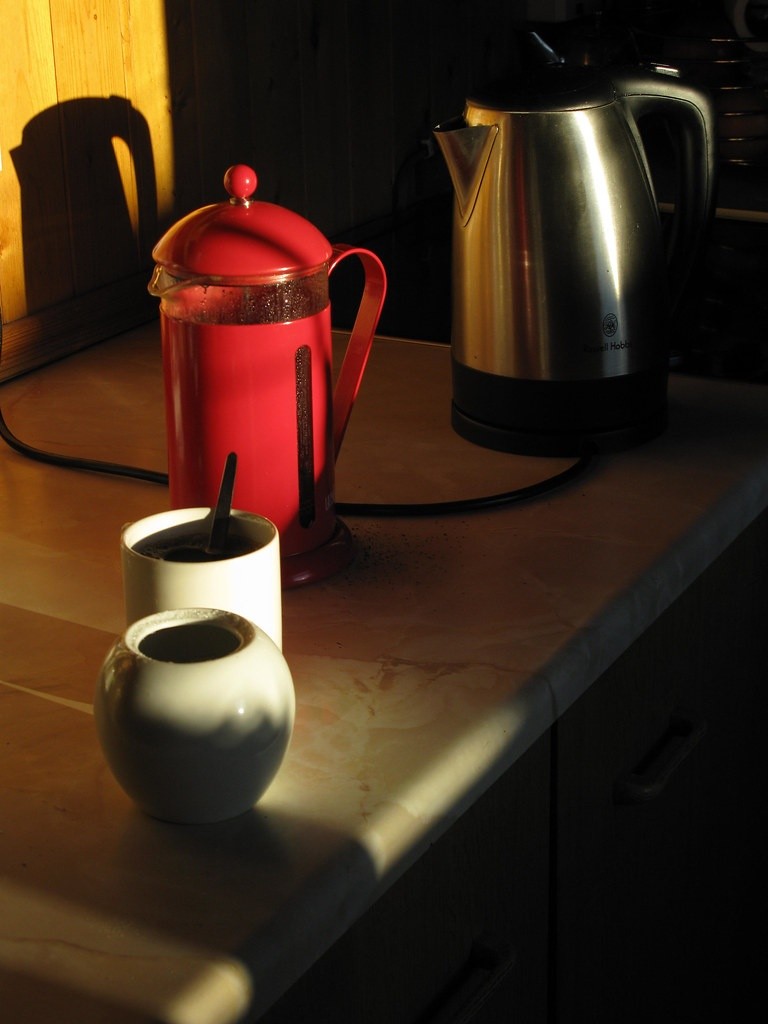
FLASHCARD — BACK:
[94,609,294,822]
[121,507,282,652]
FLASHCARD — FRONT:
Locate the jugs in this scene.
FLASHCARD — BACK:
[147,165,387,588]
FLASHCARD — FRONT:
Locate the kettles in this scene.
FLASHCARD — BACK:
[434,64,716,457]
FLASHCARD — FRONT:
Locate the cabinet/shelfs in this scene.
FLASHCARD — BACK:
[1,317,767,1024]
